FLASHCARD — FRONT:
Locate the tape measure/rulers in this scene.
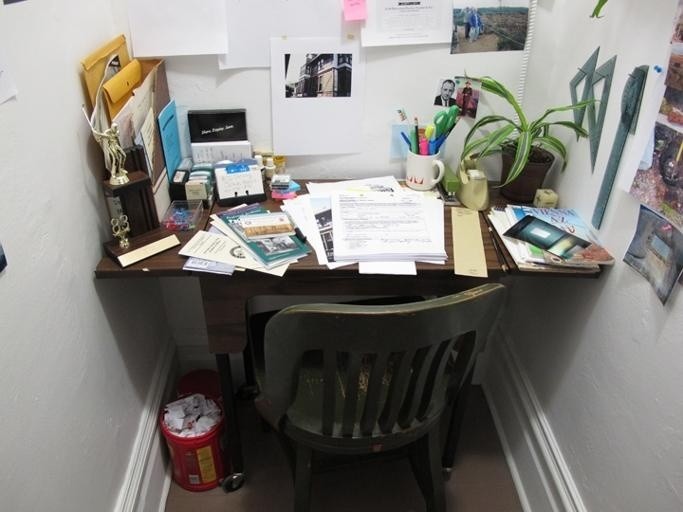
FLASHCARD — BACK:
[591,65,649,230]
[570,47,599,142]
[587,54,617,174]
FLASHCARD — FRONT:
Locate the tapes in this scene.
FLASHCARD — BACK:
[467,169,481,180]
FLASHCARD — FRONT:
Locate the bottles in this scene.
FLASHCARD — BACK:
[254,155,287,178]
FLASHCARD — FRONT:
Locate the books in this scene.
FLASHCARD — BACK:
[178,203,312,281]
[482,202,616,277]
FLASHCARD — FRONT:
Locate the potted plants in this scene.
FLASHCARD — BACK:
[460,70,602,206]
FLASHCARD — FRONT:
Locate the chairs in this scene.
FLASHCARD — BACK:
[240,280,507,510]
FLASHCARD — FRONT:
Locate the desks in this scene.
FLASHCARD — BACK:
[91,168,611,496]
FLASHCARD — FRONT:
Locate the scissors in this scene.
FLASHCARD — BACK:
[430,105,459,143]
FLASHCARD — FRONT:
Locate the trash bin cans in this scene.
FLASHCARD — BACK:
[159,388,225,491]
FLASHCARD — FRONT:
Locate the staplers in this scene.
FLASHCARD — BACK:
[435,164,462,206]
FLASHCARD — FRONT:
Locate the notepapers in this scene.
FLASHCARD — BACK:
[271,180,301,199]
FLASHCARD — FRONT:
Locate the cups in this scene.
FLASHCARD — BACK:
[404,145,444,191]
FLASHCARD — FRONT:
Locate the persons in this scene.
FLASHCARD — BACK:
[460,8,473,38]
[468,7,484,43]
[434,80,457,106]
[97,123,127,180]
[460,79,473,118]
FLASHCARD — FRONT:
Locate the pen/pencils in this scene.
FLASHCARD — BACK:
[284,209,307,242]
[488,227,509,272]
[396,109,460,155]
